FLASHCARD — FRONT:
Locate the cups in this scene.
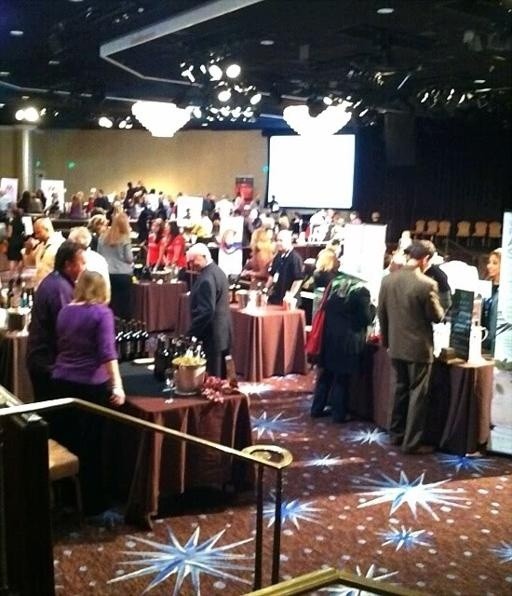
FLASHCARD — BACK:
[282,297,297,311]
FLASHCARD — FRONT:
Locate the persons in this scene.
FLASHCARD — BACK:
[0,179,501,527]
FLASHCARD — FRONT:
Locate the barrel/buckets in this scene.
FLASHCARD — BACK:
[171,356,207,397]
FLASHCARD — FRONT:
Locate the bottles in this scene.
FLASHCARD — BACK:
[7,279,35,309]
[112,316,206,383]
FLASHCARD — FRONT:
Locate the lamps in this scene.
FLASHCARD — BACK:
[322,93,370,119]
[207,52,262,123]
[13,102,61,125]
[417,85,466,110]
[99,116,133,130]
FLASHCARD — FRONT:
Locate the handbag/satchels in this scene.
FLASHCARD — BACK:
[304,307,325,356]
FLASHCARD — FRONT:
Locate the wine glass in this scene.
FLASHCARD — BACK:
[164,367,178,403]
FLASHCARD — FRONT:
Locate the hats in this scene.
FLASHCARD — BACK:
[338,261,368,282]
